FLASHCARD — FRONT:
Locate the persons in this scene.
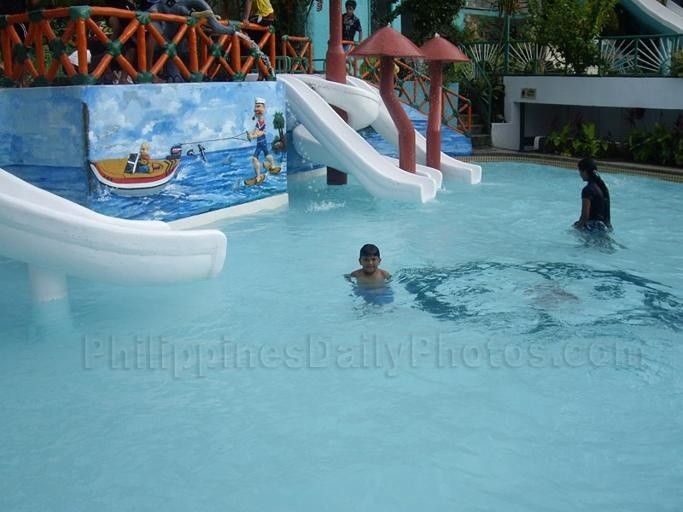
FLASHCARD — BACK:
[342,244,393,305]
[571,158,611,233]
[340,0,362,53]
[241,1,275,45]
[93,1,136,84]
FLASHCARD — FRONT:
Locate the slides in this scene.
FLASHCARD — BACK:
[276,70,482,204]
[0,168,227,285]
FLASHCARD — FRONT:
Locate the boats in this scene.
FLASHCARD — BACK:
[88,146,181,189]
